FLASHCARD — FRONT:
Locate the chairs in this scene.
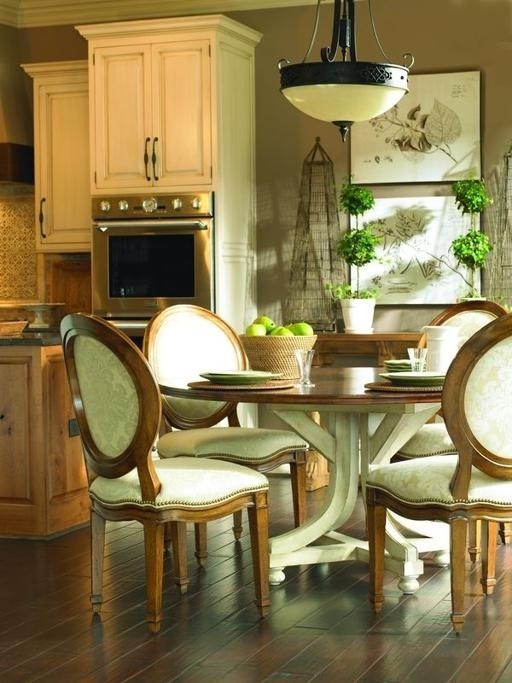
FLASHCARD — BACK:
[58,309,279,636]
[139,300,309,566]
[366,305,511,639]
[390,297,512,563]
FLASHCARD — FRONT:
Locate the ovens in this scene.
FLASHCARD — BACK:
[89,192,214,320]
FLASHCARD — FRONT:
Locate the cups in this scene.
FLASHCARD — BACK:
[424,325,457,372]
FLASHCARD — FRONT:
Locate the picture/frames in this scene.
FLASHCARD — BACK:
[347,193,487,310]
[346,67,486,187]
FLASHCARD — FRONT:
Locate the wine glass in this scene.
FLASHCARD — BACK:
[296,348,317,389]
[406,347,426,373]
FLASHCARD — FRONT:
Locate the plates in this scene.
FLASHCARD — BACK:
[381,372,448,384]
[199,370,281,384]
[384,359,426,372]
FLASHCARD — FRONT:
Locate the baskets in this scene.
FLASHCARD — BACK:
[238,334,317,381]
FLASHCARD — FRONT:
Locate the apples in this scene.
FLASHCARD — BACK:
[246,316,314,337]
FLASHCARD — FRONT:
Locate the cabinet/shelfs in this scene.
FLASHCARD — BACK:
[73,13,264,194]
[0,340,92,542]
[18,56,93,255]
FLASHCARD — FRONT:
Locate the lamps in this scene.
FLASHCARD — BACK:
[275,0,420,145]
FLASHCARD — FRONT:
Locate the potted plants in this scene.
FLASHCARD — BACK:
[325,183,384,334]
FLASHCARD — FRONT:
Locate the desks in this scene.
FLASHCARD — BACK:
[304,329,426,368]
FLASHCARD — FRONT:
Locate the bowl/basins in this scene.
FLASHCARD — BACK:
[0,320,29,338]
